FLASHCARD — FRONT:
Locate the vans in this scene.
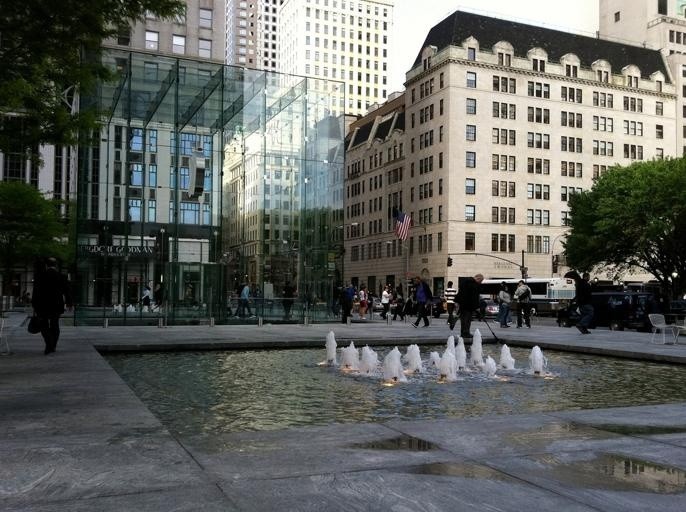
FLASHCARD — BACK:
[556,291,664,333]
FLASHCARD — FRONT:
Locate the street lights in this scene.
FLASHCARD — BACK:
[160,228,165,306]
[304,178,311,235]
[320,213,328,242]
[102,225,110,318]
[386,241,409,297]
[213,231,219,319]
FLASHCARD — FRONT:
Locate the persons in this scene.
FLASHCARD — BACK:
[479,299,487,322]
[154,283,162,306]
[142,285,152,310]
[280,280,296,316]
[494,280,532,328]
[32,256,74,354]
[454,273,484,337]
[444,280,457,325]
[575,272,595,334]
[341,275,433,328]
[231,281,262,319]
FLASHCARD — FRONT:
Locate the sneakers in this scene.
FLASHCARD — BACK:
[501,324,531,329]
[358,315,367,320]
[461,333,474,338]
[411,322,430,328]
[575,323,592,334]
[44,345,57,355]
[379,313,404,321]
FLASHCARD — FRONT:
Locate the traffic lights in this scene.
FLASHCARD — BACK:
[343,223,358,286]
[553,261,558,273]
[447,257,452,267]
[323,226,343,279]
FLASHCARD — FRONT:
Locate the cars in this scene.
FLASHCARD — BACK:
[391,295,447,318]
[305,297,327,310]
[335,294,362,311]
[477,299,499,318]
[366,297,385,310]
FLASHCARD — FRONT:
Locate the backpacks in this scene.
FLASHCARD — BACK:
[519,290,530,301]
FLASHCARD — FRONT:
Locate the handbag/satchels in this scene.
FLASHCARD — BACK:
[494,291,504,305]
[27,311,41,335]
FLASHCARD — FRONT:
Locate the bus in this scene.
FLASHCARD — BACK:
[477,278,577,317]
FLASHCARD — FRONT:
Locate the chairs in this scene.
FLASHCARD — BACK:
[647,313,684,345]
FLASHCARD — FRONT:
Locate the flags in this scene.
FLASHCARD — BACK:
[395,210,411,241]
